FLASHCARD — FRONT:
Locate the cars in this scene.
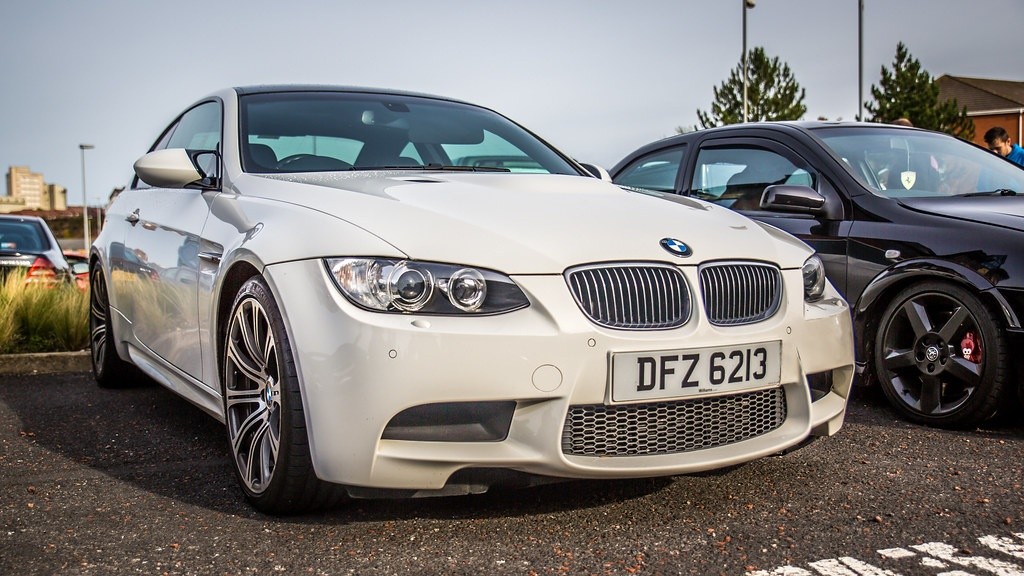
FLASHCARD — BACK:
[87,83,855,520]
[0,214,76,291]
[606,120,1024,428]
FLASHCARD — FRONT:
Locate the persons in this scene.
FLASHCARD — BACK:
[975,126,1024,194]
[886,117,942,198]
